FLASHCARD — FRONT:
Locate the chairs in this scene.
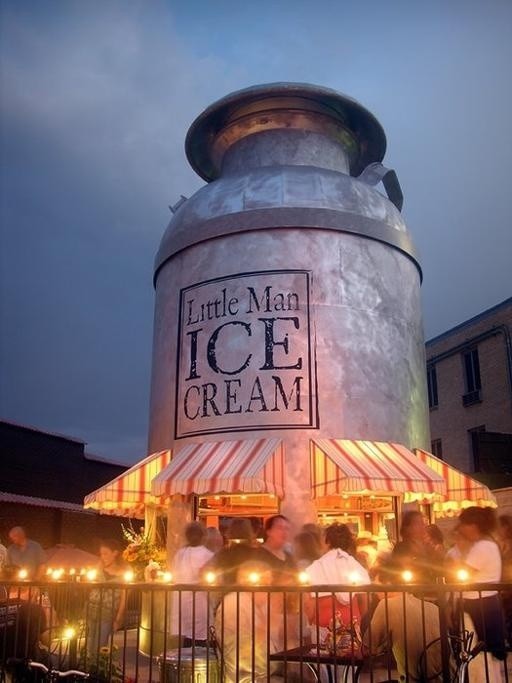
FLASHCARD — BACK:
[410,630,477,683]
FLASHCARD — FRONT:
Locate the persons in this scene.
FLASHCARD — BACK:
[1,505,511,682]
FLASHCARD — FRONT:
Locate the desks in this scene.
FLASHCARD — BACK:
[270,643,381,683]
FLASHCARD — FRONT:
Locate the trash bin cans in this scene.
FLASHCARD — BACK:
[158,648,224,683]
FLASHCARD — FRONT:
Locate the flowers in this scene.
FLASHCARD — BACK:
[114,521,158,564]
